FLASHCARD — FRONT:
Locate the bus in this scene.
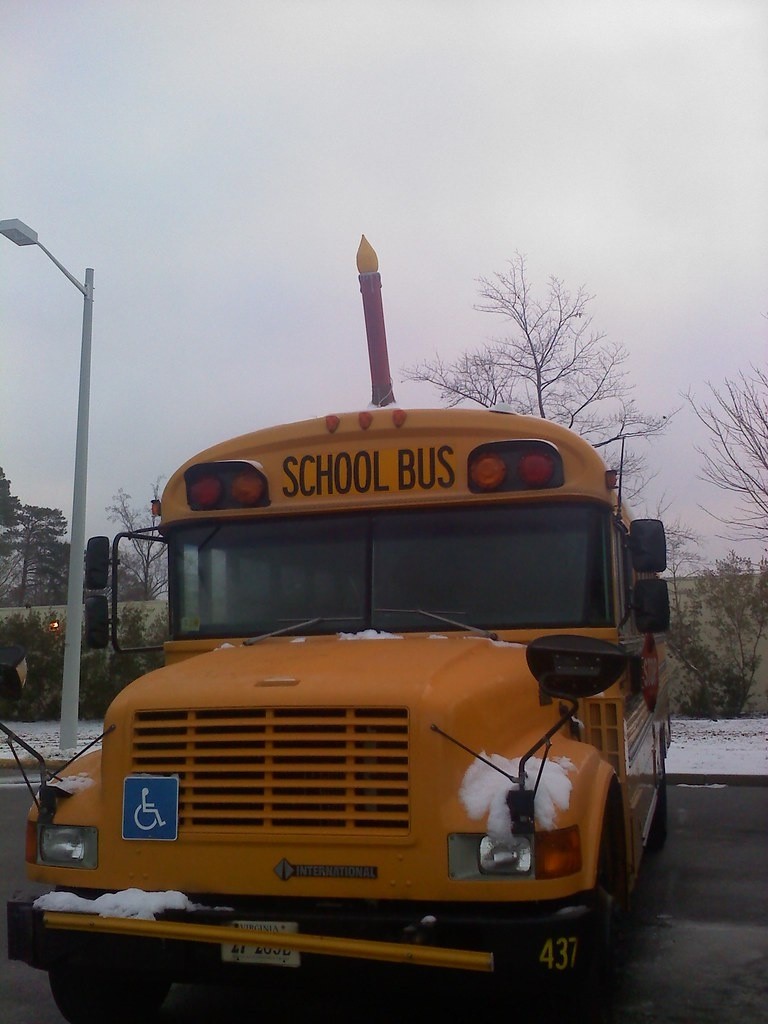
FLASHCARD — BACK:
[8,405,672,1017]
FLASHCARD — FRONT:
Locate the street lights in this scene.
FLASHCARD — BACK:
[1,217,94,751]
